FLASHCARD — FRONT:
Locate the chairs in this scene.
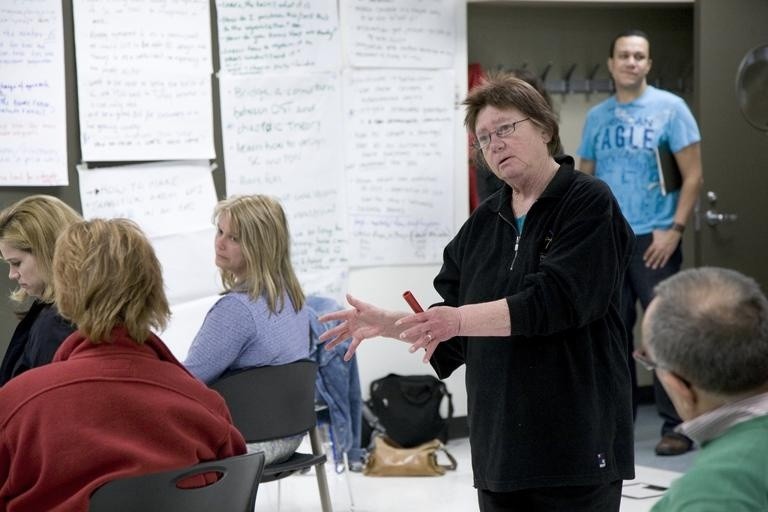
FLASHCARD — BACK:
[87,452,267,511]
[209,360,334,511]
[304,296,355,506]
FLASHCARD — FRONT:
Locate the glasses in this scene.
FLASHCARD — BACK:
[470,117,530,150]
[632,349,691,387]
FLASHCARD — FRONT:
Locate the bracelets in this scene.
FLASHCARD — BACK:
[669,222,686,232]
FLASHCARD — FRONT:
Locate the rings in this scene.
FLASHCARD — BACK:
[427,334,434,342]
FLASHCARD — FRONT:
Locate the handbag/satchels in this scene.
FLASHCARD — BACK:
[364,431,457,477]
[360,371,454,465]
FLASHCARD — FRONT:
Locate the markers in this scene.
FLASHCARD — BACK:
[403,291,429,313]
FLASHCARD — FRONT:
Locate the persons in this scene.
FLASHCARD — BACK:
[1,193,81,388]
[179,194,316,468]
[318,73,639,511]
[643,267,768,512]
[0,218,248,512]
[577,30,702,455]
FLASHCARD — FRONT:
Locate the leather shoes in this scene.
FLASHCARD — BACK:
[656,433,692,455]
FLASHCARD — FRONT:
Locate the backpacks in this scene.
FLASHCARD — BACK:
[361,371,456,450]
